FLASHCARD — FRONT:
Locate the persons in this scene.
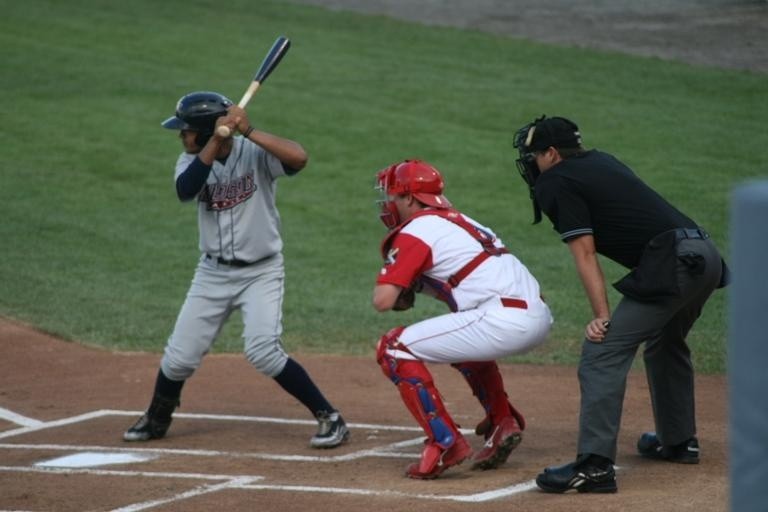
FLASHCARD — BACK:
[368,159,559,480]
[123,90,351,449]
[510,112,733,496]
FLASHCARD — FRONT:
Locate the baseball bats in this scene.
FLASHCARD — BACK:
[216,36,290,137]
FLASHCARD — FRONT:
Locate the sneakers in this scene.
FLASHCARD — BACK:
[638,432,699,464]
[310,410,350,448]
[405,431,475,480]
[470,416,522,471]
[536,462,617,493]
[123,411,172,441]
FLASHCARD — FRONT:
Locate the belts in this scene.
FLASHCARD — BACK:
[207,254,272,267]
[501,296,545,308]
[676,228,709,239]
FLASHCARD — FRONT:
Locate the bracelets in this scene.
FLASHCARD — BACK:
[243,126,254,137]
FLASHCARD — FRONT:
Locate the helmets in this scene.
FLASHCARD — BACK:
[512,114,582,226]
[374,159,452,230]
[161,91,234,148]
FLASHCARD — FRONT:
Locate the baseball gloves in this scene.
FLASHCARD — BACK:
[392,288,415,312]
